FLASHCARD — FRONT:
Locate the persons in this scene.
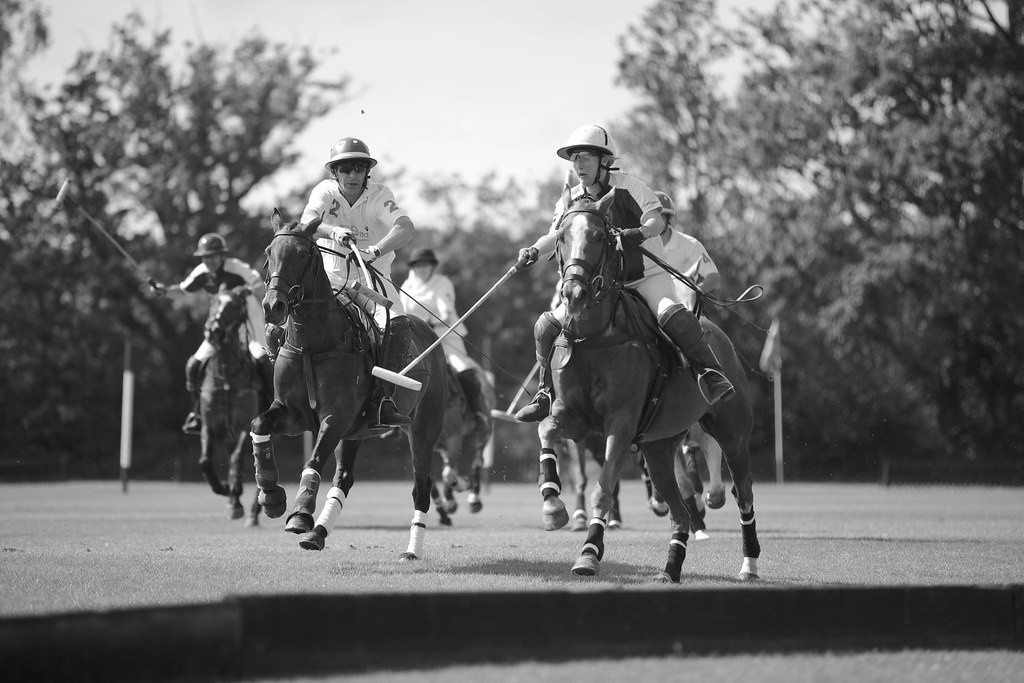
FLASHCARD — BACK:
[514,124,737,424]
[399,247,494,433]
[250,136,428,437]
[147,232,273,433]
[641,190,716,310]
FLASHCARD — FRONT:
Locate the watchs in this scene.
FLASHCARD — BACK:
[368,245,381,257]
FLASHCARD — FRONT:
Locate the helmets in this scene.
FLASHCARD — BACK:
[193,233,228,257]
[407,248,439,268]
[557,125,616,160]
[654,191,675,215]
[325,138,378,171]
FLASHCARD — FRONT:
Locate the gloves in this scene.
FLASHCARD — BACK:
[328,227,356,248]
[518,247,539,267]
[620,228,648,248]
[346,248,376,267]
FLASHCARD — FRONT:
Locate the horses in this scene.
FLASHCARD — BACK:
[249,208,449,560]
[431,370,490,527]
[535,182,763,581]
[197,290,263,528]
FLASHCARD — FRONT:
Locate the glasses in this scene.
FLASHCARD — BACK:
[569,151,596,162]
[338,162,368,173]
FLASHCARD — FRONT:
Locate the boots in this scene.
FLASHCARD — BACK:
[659,304,730,398]
[456,368,491,429]
[514,312,561,422]
[368,315,412,425]
[184,356,205,431]
